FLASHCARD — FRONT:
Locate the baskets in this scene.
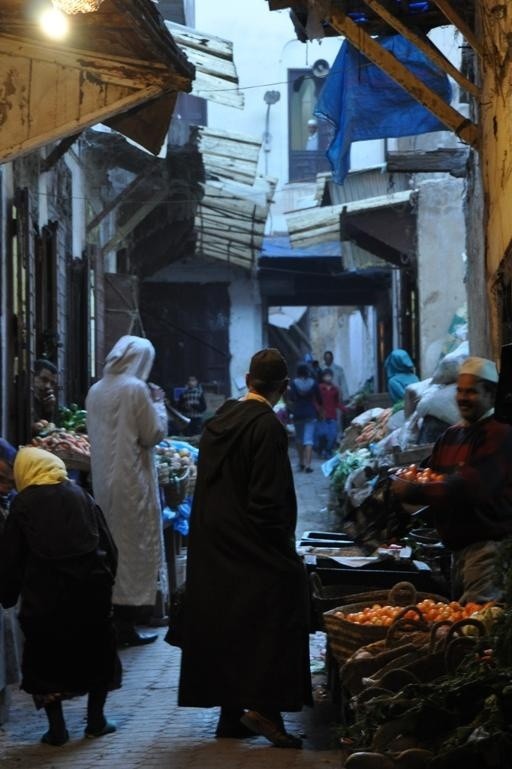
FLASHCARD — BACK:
[322,581,436,666]
[308,572,448,609]
[338,616,489,714]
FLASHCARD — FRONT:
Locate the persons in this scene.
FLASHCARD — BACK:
[32,357,57,437]
[164,348,315,749]
[388,356,512,602]
[86,334,169,647]
[0,437,17,501]
[177,376,207,436]
[384,348,418,406]
[282,349,354,474]
[1,446,124,747]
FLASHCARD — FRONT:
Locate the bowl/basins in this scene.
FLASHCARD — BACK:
[387,467,441,506]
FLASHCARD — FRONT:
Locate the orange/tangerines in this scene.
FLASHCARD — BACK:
[334,599,481,626]
[395,464,444,483]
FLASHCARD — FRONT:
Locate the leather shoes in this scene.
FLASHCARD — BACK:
[239,710,303,747]
[40,727,69,745]
[114,627,159,647]
[83,718,117,737]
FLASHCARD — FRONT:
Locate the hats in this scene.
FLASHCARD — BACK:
[457,357,500,384]
